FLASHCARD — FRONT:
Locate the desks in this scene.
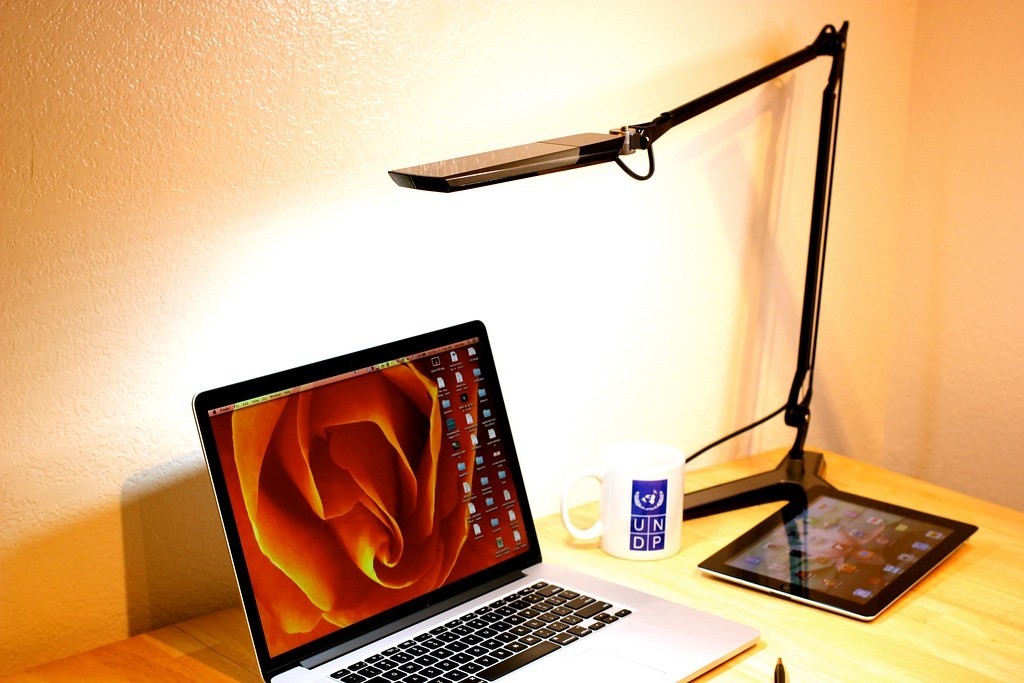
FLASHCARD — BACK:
[2,446,1024,683]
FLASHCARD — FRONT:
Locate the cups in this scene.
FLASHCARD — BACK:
[561,444,686,560]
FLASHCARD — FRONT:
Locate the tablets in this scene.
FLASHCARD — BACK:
[698,484,979,620]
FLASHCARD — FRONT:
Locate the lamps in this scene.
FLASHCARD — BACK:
[387,21,849,521]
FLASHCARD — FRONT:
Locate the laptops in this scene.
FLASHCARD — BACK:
[193,320,760,683]
